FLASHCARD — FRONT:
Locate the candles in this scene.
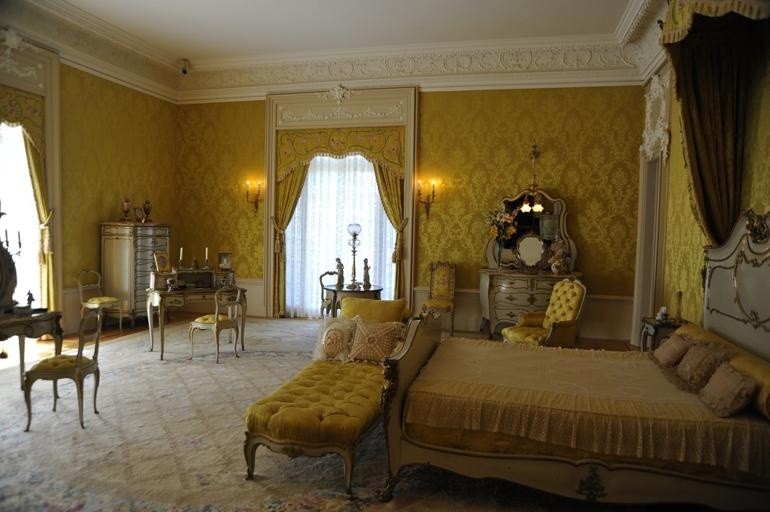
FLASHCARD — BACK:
[179,245,184,260]
[204,246,209,260]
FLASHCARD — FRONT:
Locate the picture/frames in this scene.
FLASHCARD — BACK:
[152,250,172,274]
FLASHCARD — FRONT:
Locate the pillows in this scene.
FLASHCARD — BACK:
[313,312,360,363]
[674,322,770,423]
[649,331,695,366]
[347,318,407,365]
[697,362,763,417]
[676,338,742,389]
[338,293,412,324]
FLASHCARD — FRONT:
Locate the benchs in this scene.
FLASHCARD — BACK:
[240,294,420,495]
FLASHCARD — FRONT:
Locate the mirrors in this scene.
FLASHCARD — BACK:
[488,182,577,273]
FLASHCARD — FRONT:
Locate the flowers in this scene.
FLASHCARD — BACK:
[488,205,518,242]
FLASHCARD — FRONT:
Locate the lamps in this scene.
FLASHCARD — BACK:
[520,190,532,214]
[346,219,362,285]
[532,192,544,215]
[380,204,770,512]
[415,178,441,222]
[245,178,266,220]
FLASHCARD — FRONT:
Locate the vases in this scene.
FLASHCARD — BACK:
[495,239,503,271]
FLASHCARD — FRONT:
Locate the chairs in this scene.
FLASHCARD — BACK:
[421,259,457,337]
[502,277,588,349]
[22,301,107,433]
[72,263,124,342]
[318,270,345,321]
[189,283,246,365]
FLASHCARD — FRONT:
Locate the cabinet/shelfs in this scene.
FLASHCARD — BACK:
[99,218,172,331]
[479,267,586,340]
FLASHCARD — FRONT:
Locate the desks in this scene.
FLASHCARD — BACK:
[0,307,65,413]
[320,282,384,322]
[142,268,249,362]
[636,316,695,354]
